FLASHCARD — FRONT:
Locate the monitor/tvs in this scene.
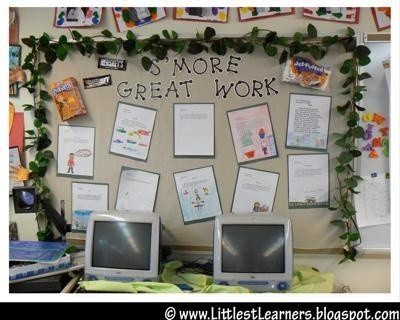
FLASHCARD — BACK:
[84,211,161,282]
[214,214,293,293]
